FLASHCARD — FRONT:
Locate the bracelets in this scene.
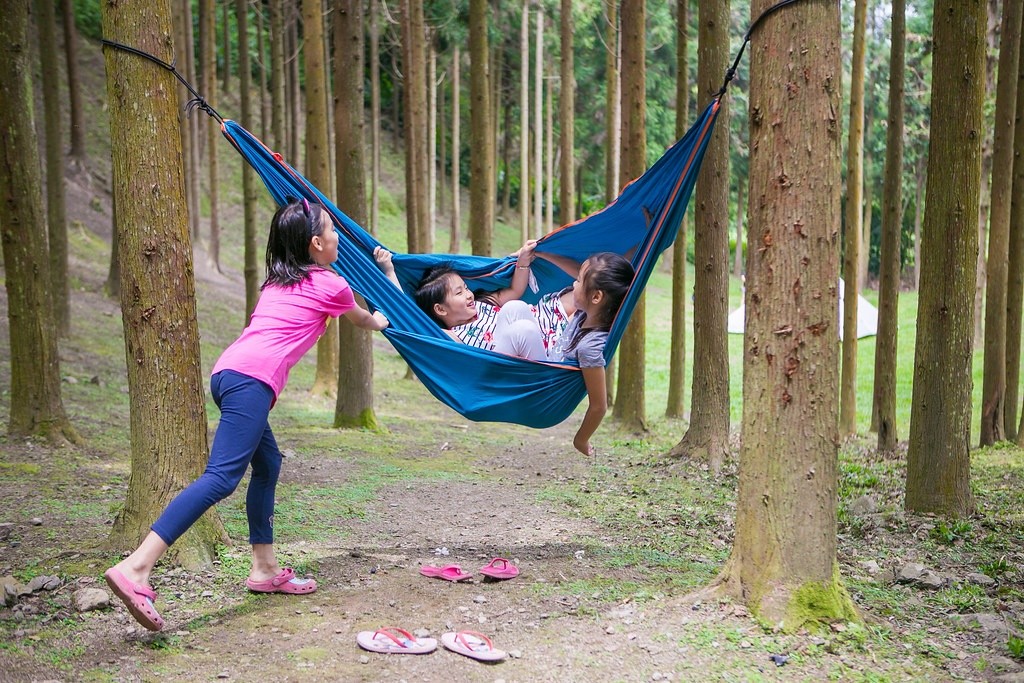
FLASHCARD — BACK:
[516,264,531,269]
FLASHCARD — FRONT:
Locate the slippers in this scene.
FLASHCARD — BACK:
[418,563,473,581]
[441,630,507,661]
[479,558,519,578]
[245,568,318,594]
[105,568,165,632]
[356,627,438,654]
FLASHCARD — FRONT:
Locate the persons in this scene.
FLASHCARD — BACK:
[372,206,672,356]
[102,197,390,630]
[492,253,636,455]
